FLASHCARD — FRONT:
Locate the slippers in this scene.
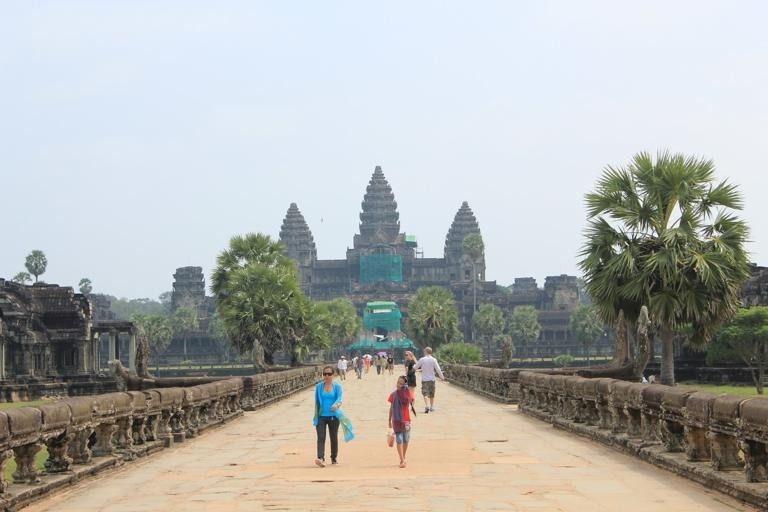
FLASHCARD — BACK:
[400,460,407,468]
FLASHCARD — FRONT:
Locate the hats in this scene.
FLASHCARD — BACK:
[422,346,433,355]
[341,356,346,358]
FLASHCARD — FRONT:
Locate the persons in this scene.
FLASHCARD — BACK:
[404,347,445,414]
[388,375,412,469]
[337,352,395,381]
[314,366,343,468]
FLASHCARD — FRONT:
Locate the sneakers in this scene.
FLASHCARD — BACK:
[424,407,435,413]
[315,458,338,467]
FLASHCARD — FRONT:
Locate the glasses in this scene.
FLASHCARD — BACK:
[322,372,334,376]
[404,354,409,357]
